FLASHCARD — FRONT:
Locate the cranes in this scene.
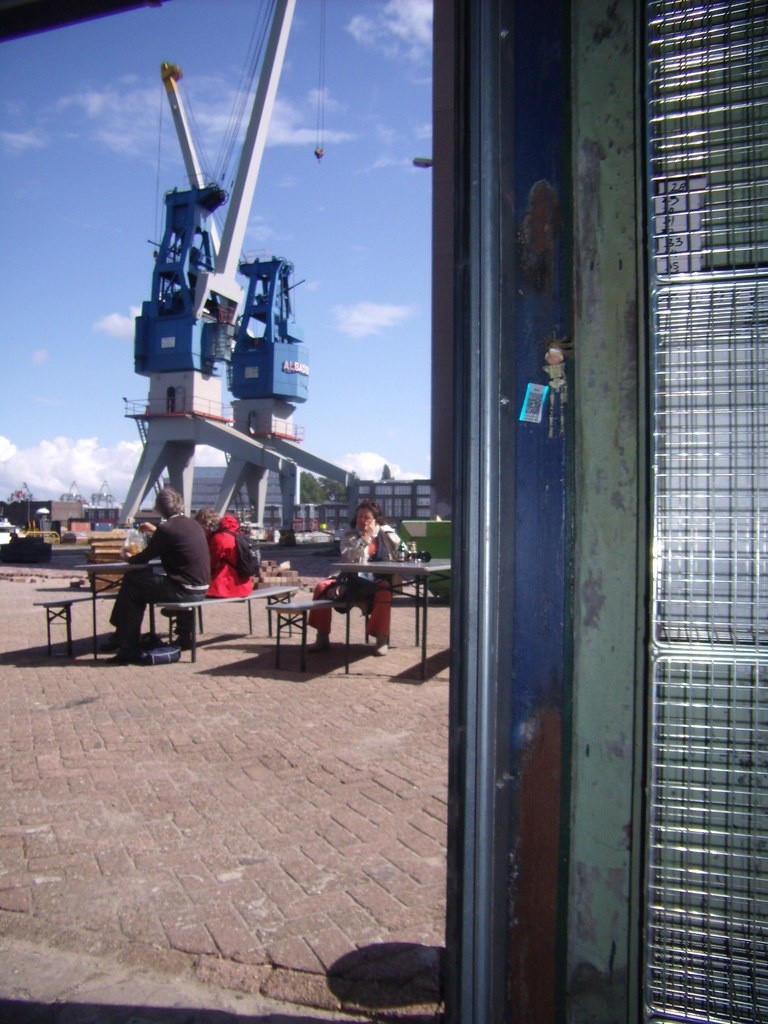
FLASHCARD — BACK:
[114,1,365,547]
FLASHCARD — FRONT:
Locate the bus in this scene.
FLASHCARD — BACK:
[26,531,61,546]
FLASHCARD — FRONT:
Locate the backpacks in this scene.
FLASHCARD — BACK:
[235,533,261,578]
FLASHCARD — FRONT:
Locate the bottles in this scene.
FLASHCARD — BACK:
[395,539,418,565]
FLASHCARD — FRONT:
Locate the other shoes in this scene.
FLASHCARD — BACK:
[99,637,142,653]
[169,637,194,650]
[307,643,330,652]
[375,644,388,655]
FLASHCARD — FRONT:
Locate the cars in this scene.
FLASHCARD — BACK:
[61,532,89,544]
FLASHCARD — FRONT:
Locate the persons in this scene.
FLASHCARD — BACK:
[99,486,212,665]
[159,507,261,652]
[305,497,408,657]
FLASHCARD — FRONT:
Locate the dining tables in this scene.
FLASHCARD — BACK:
[332,557,451,681]
[74,559,203,661]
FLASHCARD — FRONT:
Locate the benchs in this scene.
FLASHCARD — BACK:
[265,599,369,673]
[33,591,154,658]
[156,586,298,663]
